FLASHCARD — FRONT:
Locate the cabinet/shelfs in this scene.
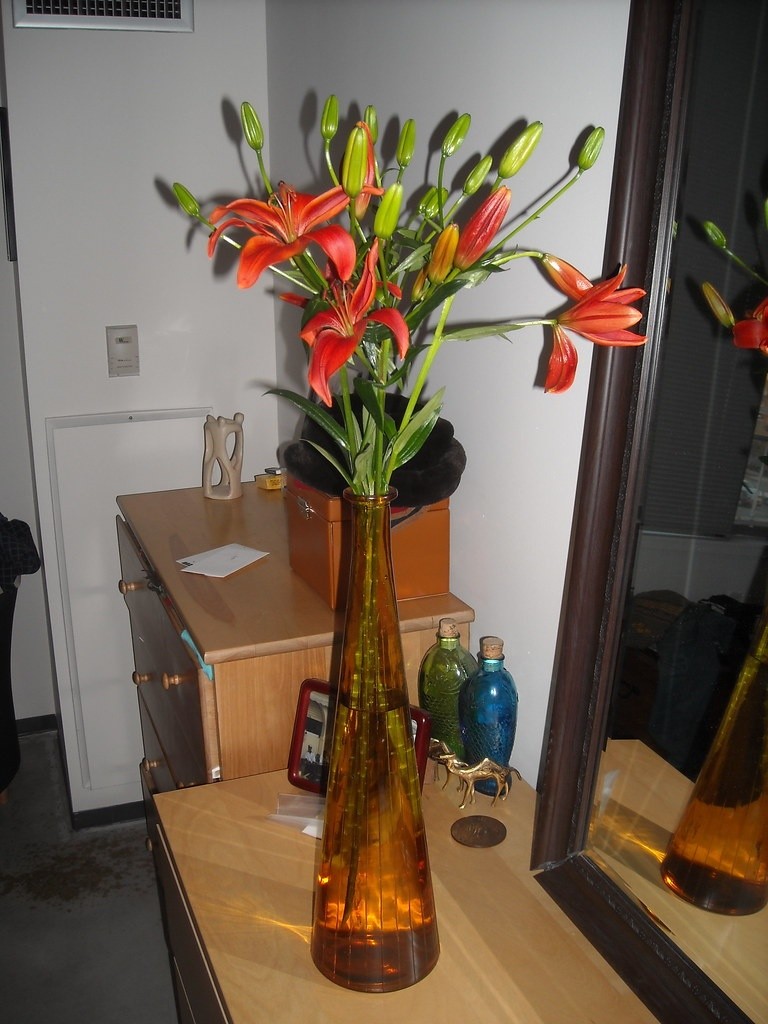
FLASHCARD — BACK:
[113,481,475,835]
[152,763,664,1024]
[586,737,768,1024]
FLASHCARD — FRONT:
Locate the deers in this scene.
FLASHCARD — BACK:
[428,738,469,782]
[446,756,524,808]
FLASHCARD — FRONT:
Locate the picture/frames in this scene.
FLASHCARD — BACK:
[288,679,431,798]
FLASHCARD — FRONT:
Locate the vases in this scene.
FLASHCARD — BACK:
[657,632,768,917]
[313,486,439,995]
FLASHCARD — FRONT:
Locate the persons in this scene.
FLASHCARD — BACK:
[304,745,322,765]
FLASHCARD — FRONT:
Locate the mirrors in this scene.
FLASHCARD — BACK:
[532,0,768,1024]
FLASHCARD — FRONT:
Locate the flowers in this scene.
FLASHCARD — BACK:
[170,91,650,919]
[697,217,768,357]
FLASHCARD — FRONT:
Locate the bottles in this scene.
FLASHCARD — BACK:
[417,633,480,766]
[460,652,520,797]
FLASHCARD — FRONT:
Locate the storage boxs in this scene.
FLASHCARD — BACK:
[283,473,449,609]
